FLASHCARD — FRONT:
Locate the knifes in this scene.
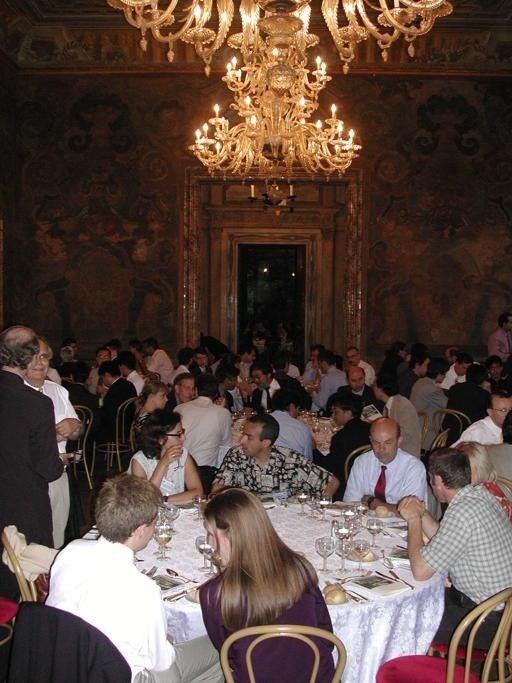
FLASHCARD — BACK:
[375,570,414,589]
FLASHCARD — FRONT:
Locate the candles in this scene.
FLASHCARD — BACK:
[248,181,260,195]
[289,185,297,196]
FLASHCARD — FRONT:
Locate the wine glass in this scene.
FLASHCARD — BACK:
[151,488,383,580]
[172,449,184,470]
[243,376,250,385]
[230,409,273,432]
[297,408,331,444]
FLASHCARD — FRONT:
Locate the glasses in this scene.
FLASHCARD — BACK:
[163,430,185,437]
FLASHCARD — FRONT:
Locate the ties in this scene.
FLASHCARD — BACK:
[265,387,271,409]
[374,466,387,504]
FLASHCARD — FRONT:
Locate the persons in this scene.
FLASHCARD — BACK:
[196,488,341,683]
[0,324,73,604]
[46,314,511,520]
[398,449,512,650]
[20,336,86,549]
[40,336,53,369]
[47,472,227,683]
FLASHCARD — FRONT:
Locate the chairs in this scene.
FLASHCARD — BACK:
[494,475,512,506]
[0,596,17,645]
[376,588,512,682]
[0,602,132,683]
[416,406,472,457]
[341,441,373,483]
[0,533,48,602]
[90,395,140,477]
[66,406,94,492]
[219,624,346,683]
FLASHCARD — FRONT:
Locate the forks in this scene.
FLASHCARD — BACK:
[326,580,368,602]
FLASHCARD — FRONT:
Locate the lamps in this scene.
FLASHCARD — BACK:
[109,0,454,187]
